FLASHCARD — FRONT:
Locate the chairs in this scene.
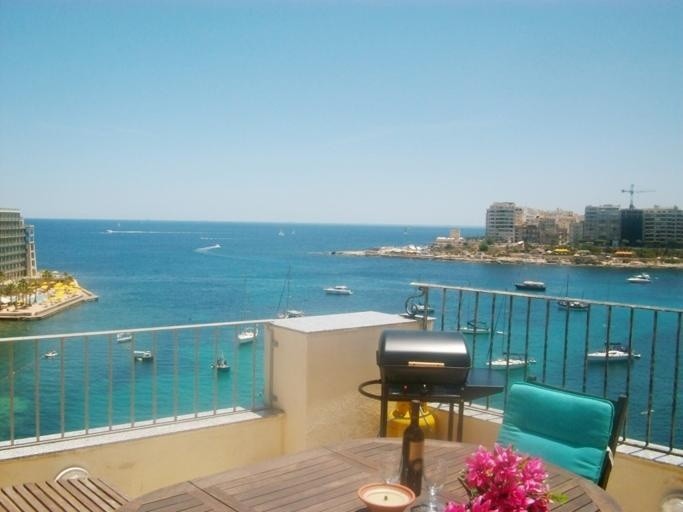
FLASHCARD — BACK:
[493,380,627,489]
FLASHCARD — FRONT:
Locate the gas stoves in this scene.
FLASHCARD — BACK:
[376,328,470,402]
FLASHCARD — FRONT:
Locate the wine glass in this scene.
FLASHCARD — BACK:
[422,460,447,512]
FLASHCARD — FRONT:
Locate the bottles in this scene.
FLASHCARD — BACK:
[400,400,424,496]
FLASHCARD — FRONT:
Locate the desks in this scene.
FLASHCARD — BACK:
[119,430,621,512]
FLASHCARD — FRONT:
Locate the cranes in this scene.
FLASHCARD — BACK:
[622,184,655,209]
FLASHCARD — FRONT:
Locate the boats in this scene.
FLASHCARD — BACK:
[626,272,655,283]
[514,280,589,311]
[116,333,153,361]
[279,309,304,319]
[45,350,58,358]
[587,341,640,364]
[456,326,537,370]
[323,285,352,295]
[210,327,258,372]
[413,303,434,314]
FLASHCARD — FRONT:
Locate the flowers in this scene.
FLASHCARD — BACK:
[444,442,568,512]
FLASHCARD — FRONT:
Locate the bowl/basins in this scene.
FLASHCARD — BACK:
[357,481,416,512]
